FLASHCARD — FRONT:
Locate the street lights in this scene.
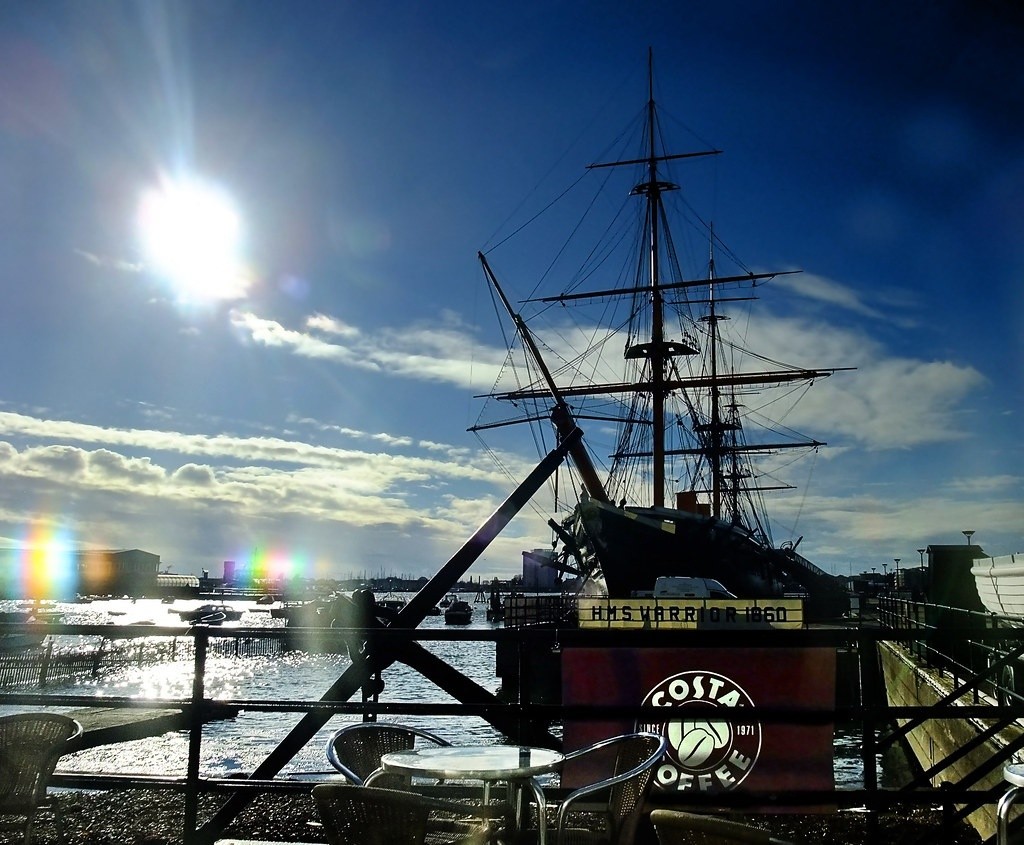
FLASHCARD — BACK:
[858,563,888,577]
[961,530,976,546]
[916,549,926,566]
[893,558,901,580]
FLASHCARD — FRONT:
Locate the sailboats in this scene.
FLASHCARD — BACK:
[469,44,860,737]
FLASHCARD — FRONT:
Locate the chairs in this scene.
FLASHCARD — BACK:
[0,713,83,845]
[311,723,667,845]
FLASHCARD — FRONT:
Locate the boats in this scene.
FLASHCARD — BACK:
[160,582,474,627]
[0,600,67,646]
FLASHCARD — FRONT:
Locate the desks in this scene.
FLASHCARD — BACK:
[996,764,1024,845]
[363,746,567,845]
